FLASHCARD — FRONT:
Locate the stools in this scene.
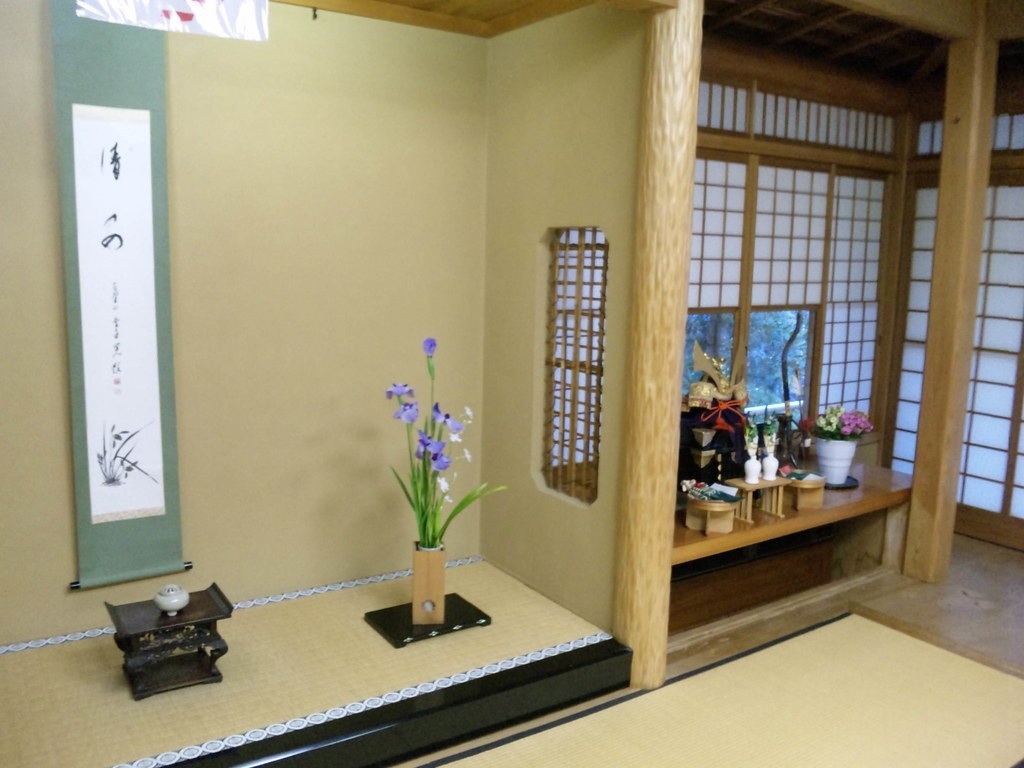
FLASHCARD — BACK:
[686,494,737,536]
[783,477,826,511]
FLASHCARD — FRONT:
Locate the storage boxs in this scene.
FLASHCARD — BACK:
[677,426,762,501]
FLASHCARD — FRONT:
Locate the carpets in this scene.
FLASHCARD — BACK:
[416,611,1024,768]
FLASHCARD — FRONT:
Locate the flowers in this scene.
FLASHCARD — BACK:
[761,404,779,452]
[741,411,758,456]
[796,406,873,443]
[384,338,506,548]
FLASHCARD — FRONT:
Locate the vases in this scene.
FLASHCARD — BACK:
[762,451,780,481]
[816,437,857,485]
[742,455,761,486]
[410,541,446,624]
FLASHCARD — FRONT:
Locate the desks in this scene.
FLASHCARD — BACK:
[724,475,790,524]
[104,581,234,702]
